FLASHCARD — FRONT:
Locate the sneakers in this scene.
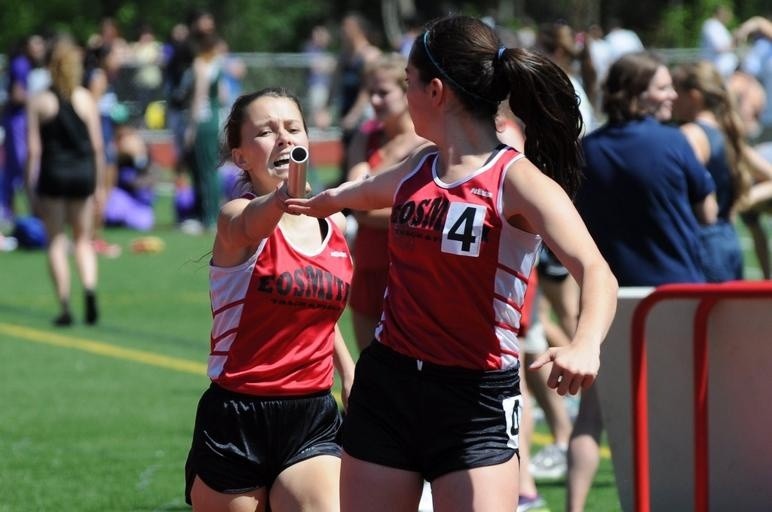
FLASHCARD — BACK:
[527,441,569,484]
[50,289,98,326]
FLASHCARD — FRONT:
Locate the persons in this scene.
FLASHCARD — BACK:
[288,16,618,512]
[182,87,355,512]
[306,13,423,182]
[0,11,245,327]
[497,5,772,512]
[345,53,426,357]
